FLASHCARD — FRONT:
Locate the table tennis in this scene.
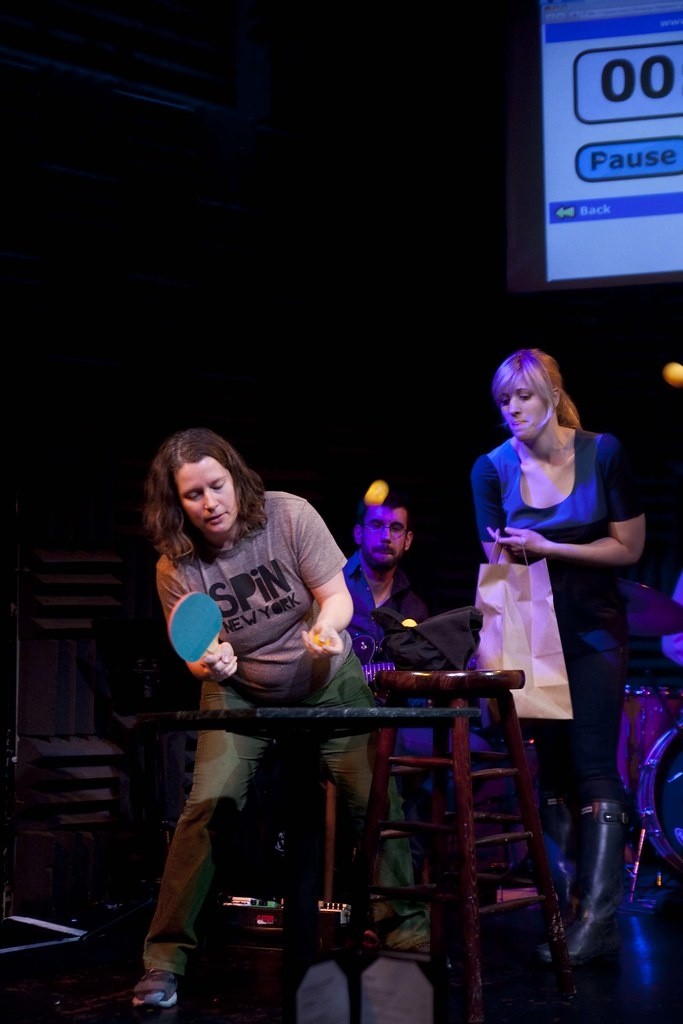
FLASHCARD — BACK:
[313,634,330,654]
[662,362,683,387]
[365,488,384,506]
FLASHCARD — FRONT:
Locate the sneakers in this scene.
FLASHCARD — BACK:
[379,941,450,965]
[134,969,178,1006]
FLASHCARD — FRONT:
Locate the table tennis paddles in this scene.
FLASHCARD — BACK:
[168,591,223,662]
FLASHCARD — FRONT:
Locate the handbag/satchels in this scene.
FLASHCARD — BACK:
[474,537,574,720]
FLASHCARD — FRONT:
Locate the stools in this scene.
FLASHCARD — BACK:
[346,670,577,1023]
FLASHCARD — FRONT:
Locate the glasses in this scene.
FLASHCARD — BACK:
[360,523,409,538]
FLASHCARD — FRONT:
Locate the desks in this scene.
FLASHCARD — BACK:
[139,708,481,949]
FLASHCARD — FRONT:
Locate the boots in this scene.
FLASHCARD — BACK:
[533,797,629,966]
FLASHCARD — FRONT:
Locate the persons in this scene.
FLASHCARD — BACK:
[297,490,492,887]
[127,427,435,1007]
[472,348,646,962]
